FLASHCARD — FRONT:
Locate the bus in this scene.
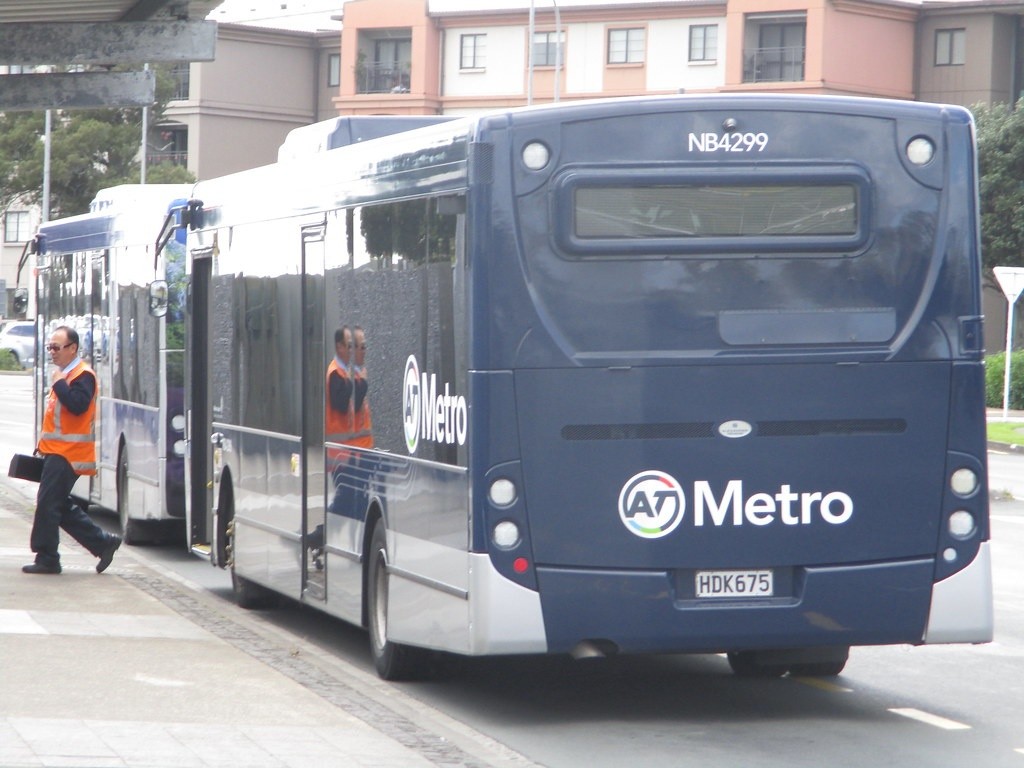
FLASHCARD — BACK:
[13,92,996,684]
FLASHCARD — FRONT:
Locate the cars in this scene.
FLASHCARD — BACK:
[0,319,37,371]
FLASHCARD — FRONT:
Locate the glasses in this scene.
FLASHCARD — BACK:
[47,341,73,352]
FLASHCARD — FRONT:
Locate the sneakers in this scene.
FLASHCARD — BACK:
[21,561,61,574]
[96,533,122,574]
[300,534,325,571]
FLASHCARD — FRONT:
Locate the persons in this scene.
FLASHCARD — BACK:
[22,325,122,574]
[299,325,373,571]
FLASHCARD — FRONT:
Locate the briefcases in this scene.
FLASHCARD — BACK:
[8,449,44,483]
